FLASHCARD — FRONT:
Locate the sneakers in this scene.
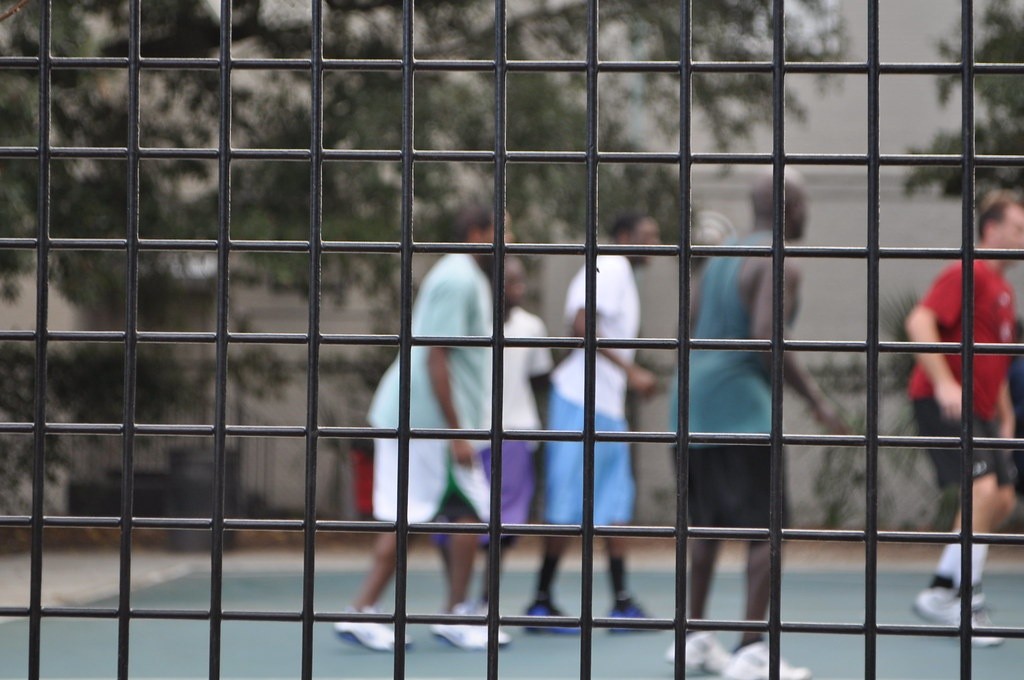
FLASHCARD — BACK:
[948,591,1002,645]
[666,624,734,678]
[721,639,812,680]
[911,577,964,621]
[430,607,499,655]
[605,605,671,634]
[521,601,595,634]
[331,606,413,654]
[469,600,514,646]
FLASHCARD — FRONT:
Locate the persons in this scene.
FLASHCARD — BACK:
[520,207,663,635]
[907,186,1024,645]
[434,248,557,648]
[331,190,516,652]
[668,167,851,680]
